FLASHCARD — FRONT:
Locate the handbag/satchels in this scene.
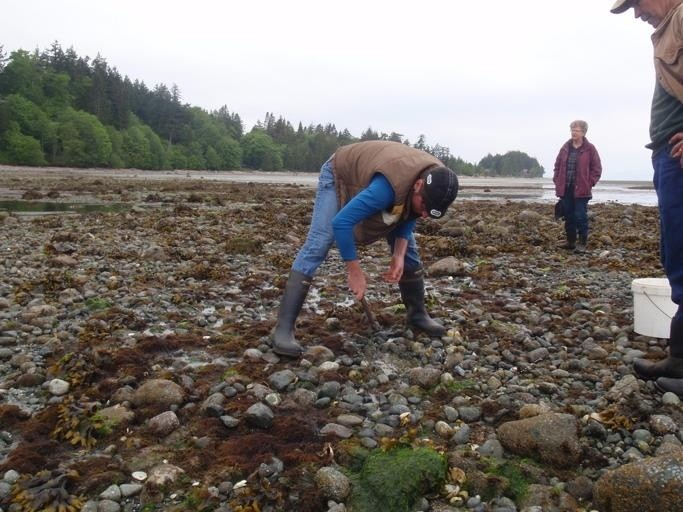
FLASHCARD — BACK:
[554,199,564,220]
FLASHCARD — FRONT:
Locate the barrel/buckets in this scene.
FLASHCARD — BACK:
[628,277,677,339]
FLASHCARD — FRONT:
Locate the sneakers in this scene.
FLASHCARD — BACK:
[557,240,590,254]
[657,378,683,395]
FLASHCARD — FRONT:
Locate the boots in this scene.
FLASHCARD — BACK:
[267,269,313,357]
[398,269,446,334]
[633,316,683,382]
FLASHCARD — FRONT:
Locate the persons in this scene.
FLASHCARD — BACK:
[552,120,603,254]
[609,0,683,399]
[267,139,460,358]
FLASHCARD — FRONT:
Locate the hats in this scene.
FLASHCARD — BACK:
[423,167,458,220]
[610,0,634,14]
